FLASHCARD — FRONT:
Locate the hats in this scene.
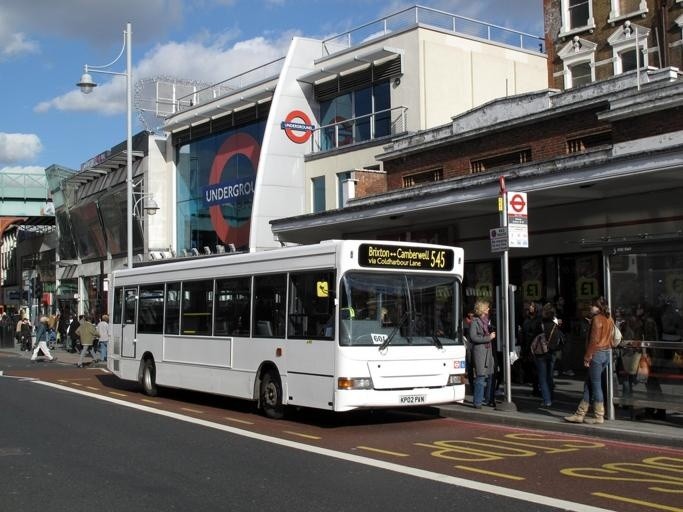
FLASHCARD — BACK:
[40,317,47,322]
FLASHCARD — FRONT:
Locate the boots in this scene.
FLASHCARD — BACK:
[584,402,605,424]
[564,399,589,423]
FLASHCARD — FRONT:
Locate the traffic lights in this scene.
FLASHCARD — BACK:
[29,279,33,296]
[36,278,42,297]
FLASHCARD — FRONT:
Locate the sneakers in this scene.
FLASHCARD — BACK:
[562,369,575,376]
[552,369,559,376]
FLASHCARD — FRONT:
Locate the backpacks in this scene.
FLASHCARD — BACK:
[531,322,557,356]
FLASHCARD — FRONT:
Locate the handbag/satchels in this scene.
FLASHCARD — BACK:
[635,335,651,383]
[622,350,642,376]
[610,322,624,348]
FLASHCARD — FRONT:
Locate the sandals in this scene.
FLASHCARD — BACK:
[535,401,552,409]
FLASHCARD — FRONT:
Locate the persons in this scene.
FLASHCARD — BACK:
[462,295,622,425]
[16,313,109,368]
[613,303,667,420]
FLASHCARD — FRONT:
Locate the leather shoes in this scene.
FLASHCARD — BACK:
[484,399,496,407]
[473,404,481,409]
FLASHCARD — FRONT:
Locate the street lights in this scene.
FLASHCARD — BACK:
[74,23,161,269]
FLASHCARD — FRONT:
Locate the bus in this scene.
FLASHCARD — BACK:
[609,251,682,341]
[102,239,466,419]
